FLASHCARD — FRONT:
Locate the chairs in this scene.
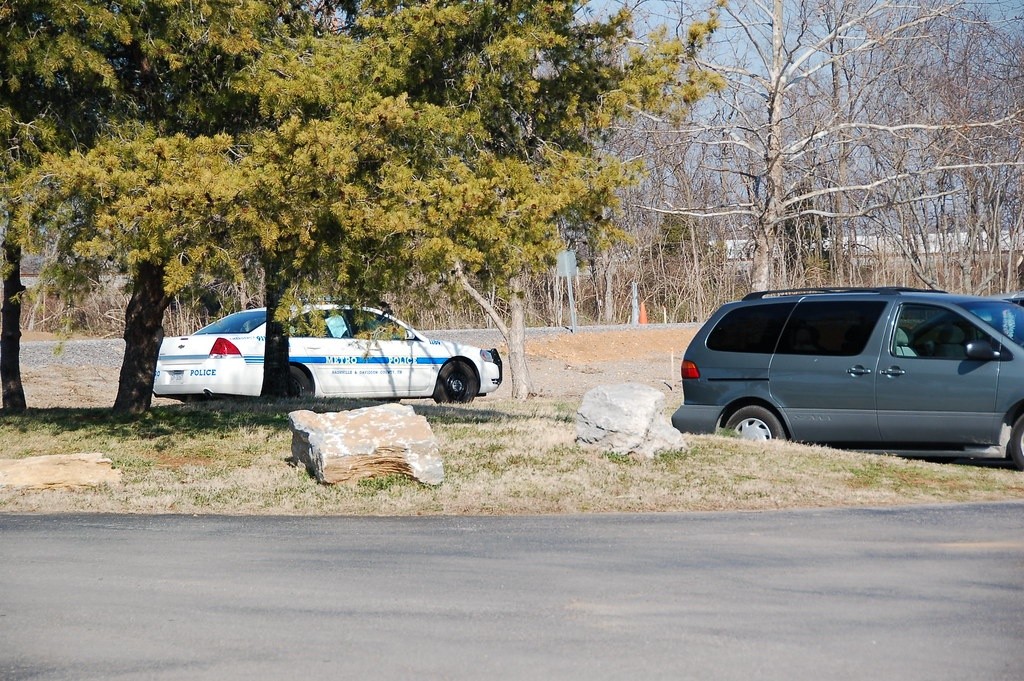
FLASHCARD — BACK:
[934,325,966,359]
[895,327,920,357]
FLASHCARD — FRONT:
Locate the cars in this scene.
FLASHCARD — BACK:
[152,304,503,404]
[908,292,1024,387]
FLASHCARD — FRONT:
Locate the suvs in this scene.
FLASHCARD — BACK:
[671,285,1024,468]
